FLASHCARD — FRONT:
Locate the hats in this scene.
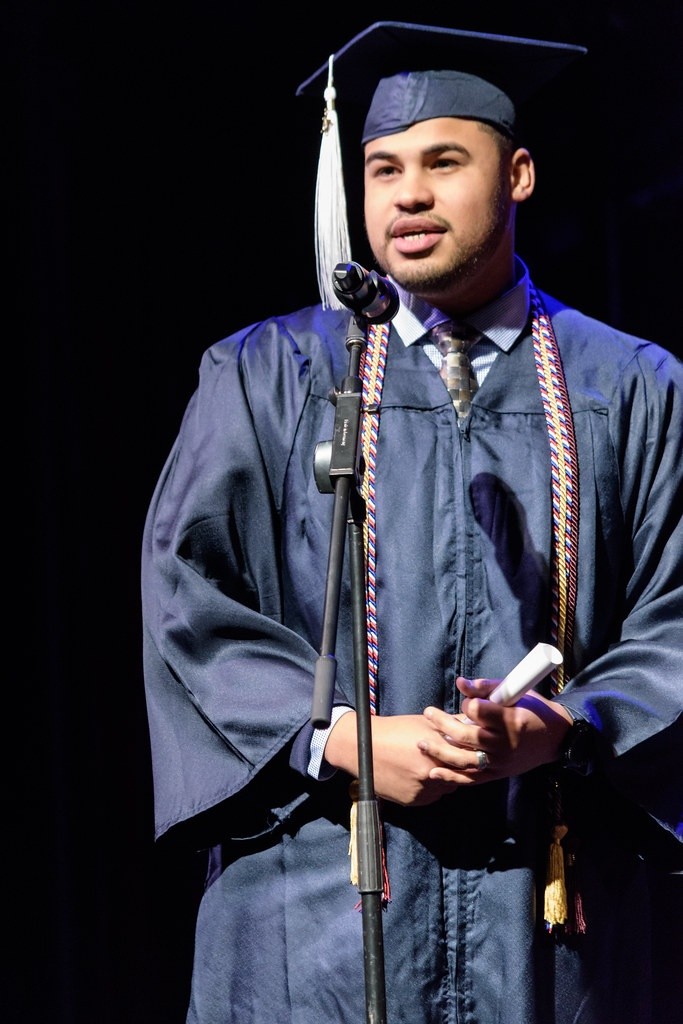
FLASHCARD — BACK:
[295,22,591,311]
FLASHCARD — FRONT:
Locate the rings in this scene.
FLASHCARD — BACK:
[475,750,488,773]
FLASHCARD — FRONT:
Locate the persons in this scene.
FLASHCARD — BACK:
[142,22,683,1024]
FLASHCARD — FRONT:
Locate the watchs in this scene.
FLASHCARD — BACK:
[554,719,600,772]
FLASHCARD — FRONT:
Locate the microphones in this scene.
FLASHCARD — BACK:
[333,263,400,325]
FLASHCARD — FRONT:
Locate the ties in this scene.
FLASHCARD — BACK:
[424,323,488,416]
